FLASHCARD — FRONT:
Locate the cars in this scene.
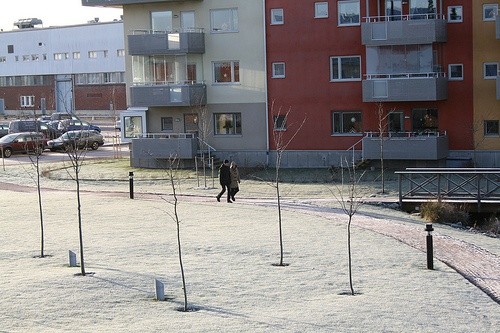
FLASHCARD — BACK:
[0,124,10,138]
[36,115,52,125]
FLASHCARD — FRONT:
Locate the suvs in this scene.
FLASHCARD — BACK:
[57,119,102,134]
[47,130,104,152]
[0,132,47,158]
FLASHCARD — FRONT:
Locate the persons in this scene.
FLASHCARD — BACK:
[228,161,241,202]
[216,158,233,203]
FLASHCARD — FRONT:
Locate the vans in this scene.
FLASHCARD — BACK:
[9,121,55,139]
[52,113,83,127]
